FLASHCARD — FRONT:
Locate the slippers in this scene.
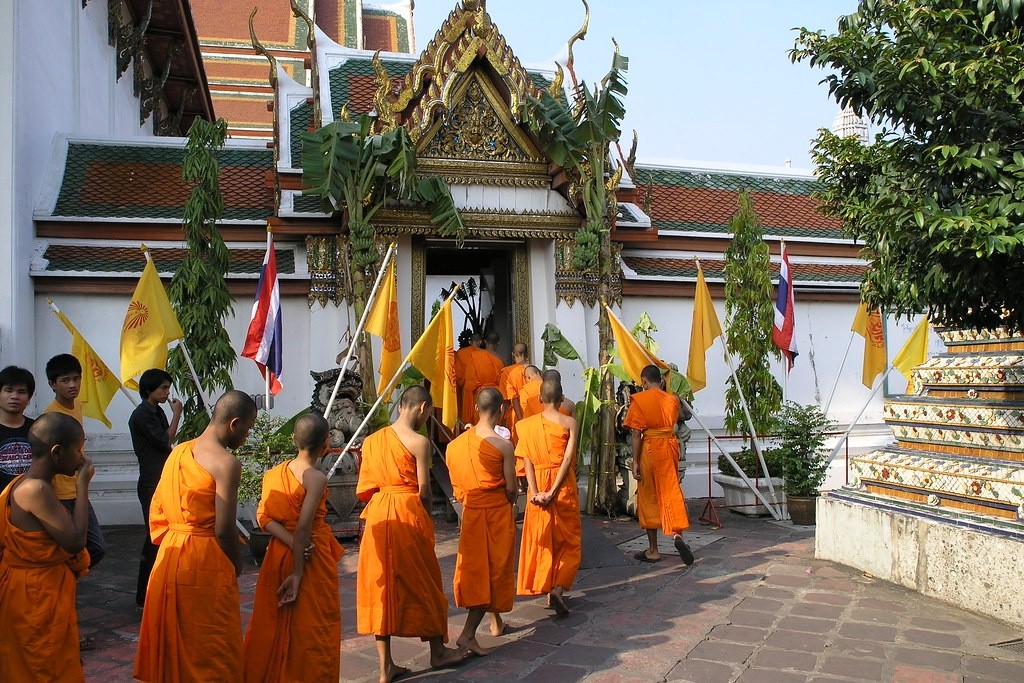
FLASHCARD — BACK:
[634,550,656,563]
[674,540,694,565]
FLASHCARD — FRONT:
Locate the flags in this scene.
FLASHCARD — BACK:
[769,244,799,373]
[120,258,184,392]
[895,313,928,394]
[58,310,122,430]
[241,231,284,397]
[405,297,458,434]
[687,267,722,394]
[850,280,887,389]
[364,255,402,403]
[607,309,670,393]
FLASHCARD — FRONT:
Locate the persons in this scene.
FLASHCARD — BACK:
[0,366,41,495]
[446,386,519,656]
[0,412,96,683]
[452,331,578,523]
[353,385,469,683]
[129,390,244,683]
[43,352,106,578]
[130,368,183,607]
[242,414,345,683]
[514,380,580,614]
[622,364,695,565]
